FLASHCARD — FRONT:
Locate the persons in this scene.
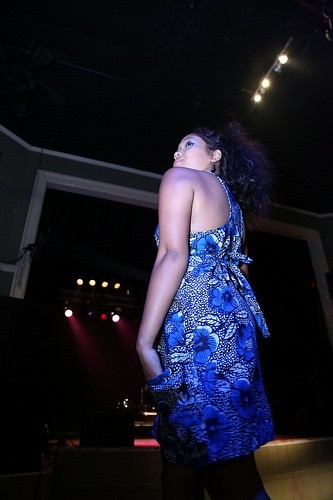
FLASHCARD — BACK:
[136,128,277,500]
